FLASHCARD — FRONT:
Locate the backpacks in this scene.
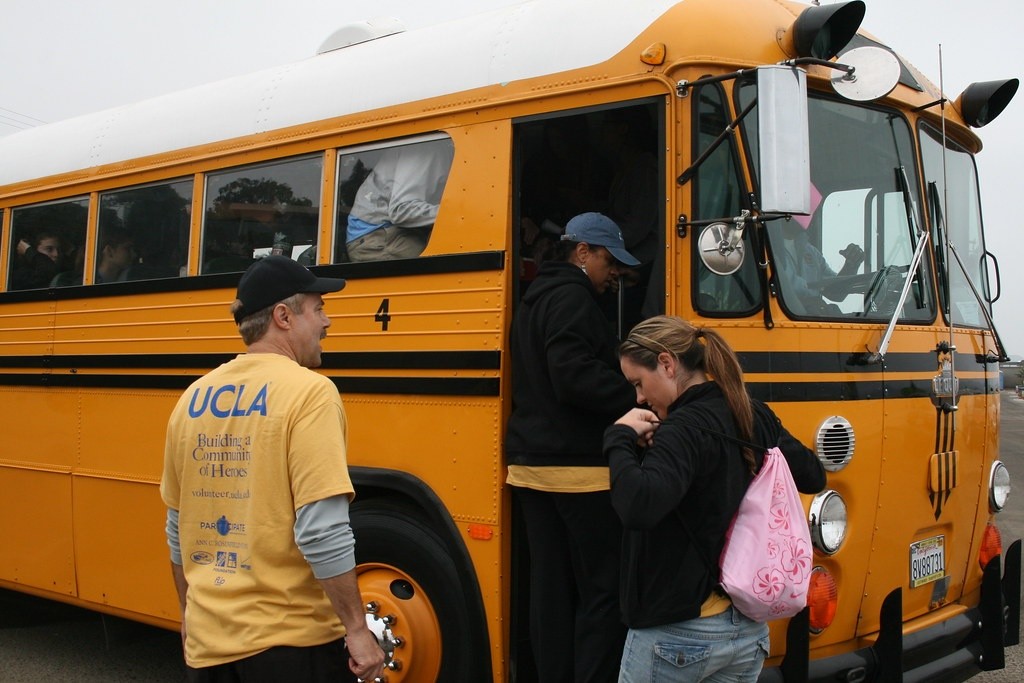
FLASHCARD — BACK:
[647,420,814,623]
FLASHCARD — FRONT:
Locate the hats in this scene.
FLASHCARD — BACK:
[561,213,642,265]
[233,256,347,324]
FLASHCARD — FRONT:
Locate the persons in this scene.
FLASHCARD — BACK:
[159,256,386,683]
[509,214,641,683]
[16,233,85,289]
[96,220,317,284]
[348,139,454,261]
[602,315,827,683]
[765,192,864,316]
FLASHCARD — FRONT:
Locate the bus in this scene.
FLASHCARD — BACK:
[0,1,1024,682]
